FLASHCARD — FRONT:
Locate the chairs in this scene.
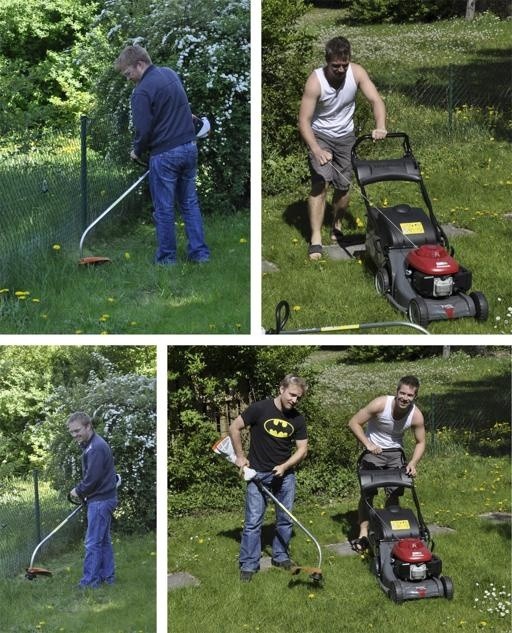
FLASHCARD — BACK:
[239,571,252,583]
[271,557,295,570]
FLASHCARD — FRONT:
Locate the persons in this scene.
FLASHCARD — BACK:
[230,374,309,582]
[115,45,210,266]
[348,376,426,548]
[298,35,388,262]
[66,411,118,588]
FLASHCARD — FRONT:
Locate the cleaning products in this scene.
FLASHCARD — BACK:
[349,447,453,602]
[350,134,489,325]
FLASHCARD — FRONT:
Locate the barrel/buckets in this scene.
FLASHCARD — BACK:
[331,224,343,241]
[309,242,322,262]
